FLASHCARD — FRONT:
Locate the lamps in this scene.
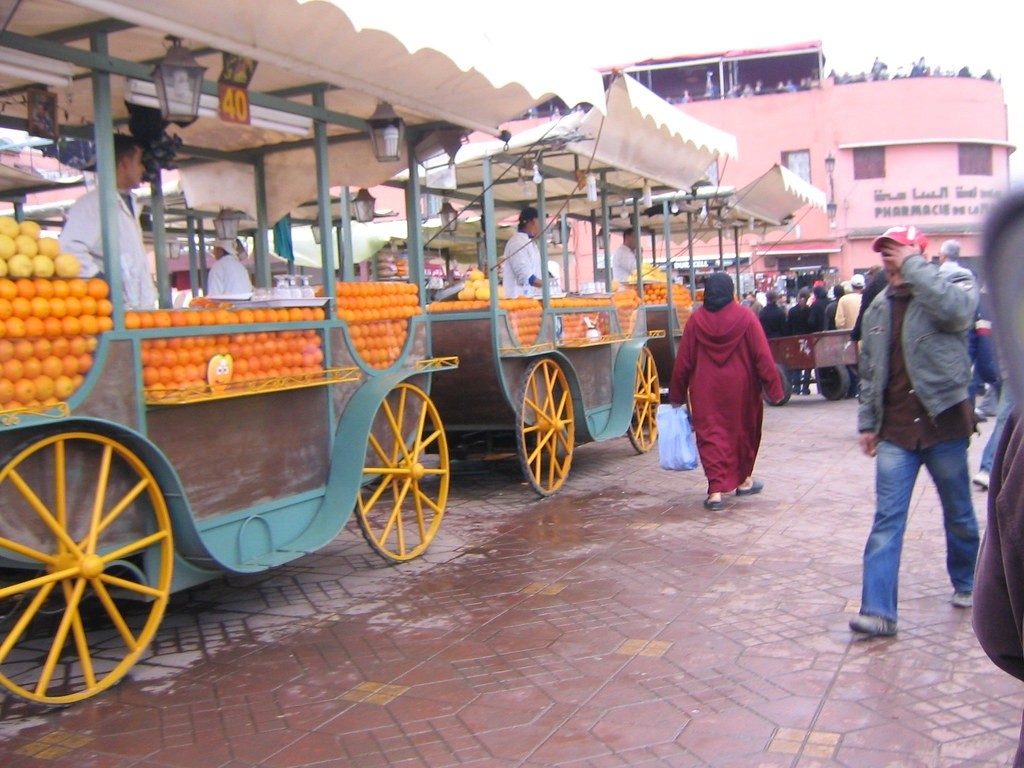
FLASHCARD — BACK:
[437,197,458,231]
[149,34,210,124]
[351,188,376,223]
[825,152,835,172]
[642,179,653,208]
[363,100,406,161]
[311,216,322,244]
[827,200,836,219]
[585,168,598,201]
[212,208,242,240]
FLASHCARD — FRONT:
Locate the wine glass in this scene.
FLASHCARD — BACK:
[534,278,562,295]
[270,274,314,300]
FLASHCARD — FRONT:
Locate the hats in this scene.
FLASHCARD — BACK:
[520,207,549,219]
[872,226,928,256]
[851,274,865,287]
[704,273,735,312]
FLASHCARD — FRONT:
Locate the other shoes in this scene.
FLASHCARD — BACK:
[792,388,811,396]
[952,591,974,608]
[736,481,763,496]
[850,614,898,636]
[704,497,722,511]
[972,472,989,489]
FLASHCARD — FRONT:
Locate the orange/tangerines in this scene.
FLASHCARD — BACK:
[317,280,423,368]
[0,276,114,414]
[427,282,644,347]
[125,297,326,398]
[640,284,690,329]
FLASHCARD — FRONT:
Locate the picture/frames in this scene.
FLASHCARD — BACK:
[26,87,59,139]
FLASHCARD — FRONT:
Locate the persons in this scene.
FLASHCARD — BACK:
[612,228,643,289]
[740,238,1014,488]
[850,227,979,637]
[972,185,1024,768]
[503,207,542,297]
[669,271,785,509]
[205,237,252,298]
[58,133,157,313]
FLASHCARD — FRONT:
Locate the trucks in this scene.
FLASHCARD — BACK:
[0,0,612,713]
[593,162,828,399]
[264,69,738,496]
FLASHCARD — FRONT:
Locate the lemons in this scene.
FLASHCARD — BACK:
[457,269,505,300]
[627,262,667,282]
[0,215,82,278]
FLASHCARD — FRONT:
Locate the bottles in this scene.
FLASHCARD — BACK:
[585,325,599,341]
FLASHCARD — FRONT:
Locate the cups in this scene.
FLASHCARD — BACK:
[579,281,606,294]
